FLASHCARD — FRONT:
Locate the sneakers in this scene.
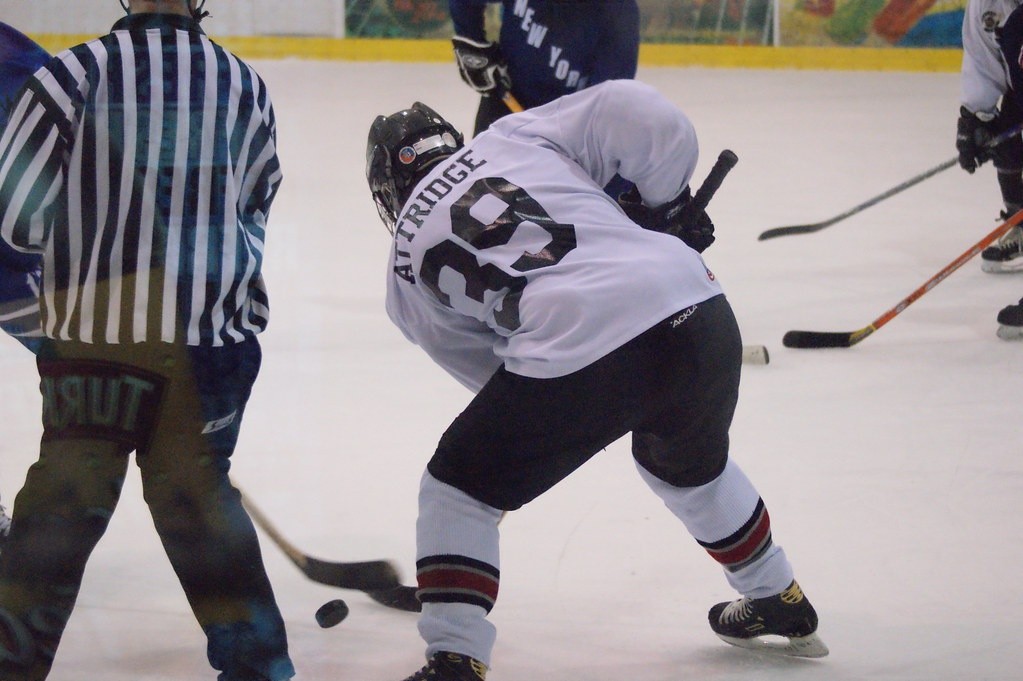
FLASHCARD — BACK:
[996,296,1023,340]
[402,650,487,681]
[709,580,829,660]
[979,210,1023,273]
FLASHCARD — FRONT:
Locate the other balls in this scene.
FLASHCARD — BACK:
[315,599,350,629]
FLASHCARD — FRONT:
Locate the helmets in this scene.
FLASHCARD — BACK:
[366,102,465,236]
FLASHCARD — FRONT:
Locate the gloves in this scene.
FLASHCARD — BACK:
[450,34,512,103]
[956,103,1002,174]
[618,181,716,254]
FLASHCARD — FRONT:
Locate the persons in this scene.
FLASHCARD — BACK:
[365,78,829,681]
[0,1,300,681]
[449,0,639,140]
[954,0,1023,340]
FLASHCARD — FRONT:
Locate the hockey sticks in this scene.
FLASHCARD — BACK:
[497,88,770,367]
[228,469,398,591]
[756,126,1023,241]
[364,150,739,613]
[783,206,1023,352]
